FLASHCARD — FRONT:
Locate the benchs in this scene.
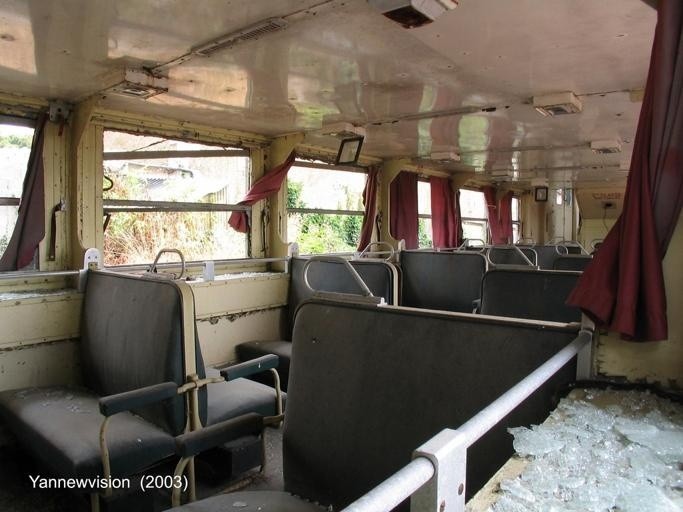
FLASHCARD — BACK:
[1,241,596,512]
